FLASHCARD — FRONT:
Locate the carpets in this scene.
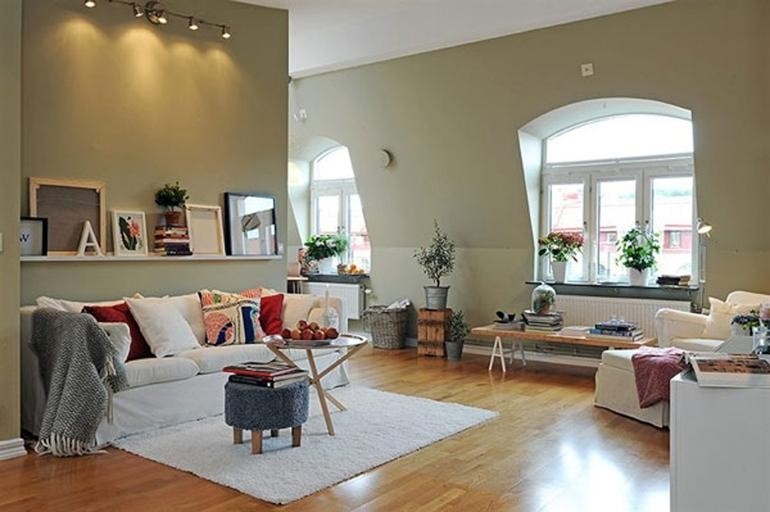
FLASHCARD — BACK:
[111,384,501,506]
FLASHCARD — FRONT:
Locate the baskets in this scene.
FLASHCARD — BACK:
[367,305,408,350]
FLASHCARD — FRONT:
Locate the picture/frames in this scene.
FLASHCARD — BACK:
[224,190,279,257]
[183,203,225,255]
[28,174,107,255]
[111,210,150,255]
[20,216,48,256]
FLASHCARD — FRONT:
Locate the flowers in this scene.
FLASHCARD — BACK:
[538,228,585,262]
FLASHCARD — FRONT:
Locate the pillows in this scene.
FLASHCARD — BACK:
[701,297,760,340]
[81,288,314,367]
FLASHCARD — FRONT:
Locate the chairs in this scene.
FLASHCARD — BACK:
[653,290,770,352]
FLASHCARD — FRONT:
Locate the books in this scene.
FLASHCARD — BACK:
[655,276,690,286]
[680,350,770,387]
[494,309,644,341]
[153,225,193,256]
[222,362,310,389]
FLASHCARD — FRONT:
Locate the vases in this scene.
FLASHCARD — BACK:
[551,262,566,283]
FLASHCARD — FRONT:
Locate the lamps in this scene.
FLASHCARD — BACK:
[84,0,232,40]
[696,216,713,310]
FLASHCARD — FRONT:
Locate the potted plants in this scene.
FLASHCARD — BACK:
[298,233,349,276]
[615,225,661,288]
[412,218,457,310]
[444,309,465,359]
[153,182,192,226]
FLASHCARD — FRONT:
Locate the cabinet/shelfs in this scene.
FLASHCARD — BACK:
[416,308,452,357]
[669,336,770,512]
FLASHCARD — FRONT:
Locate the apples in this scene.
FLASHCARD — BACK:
[281,320,338,340]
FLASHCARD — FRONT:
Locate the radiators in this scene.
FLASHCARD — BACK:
[554,294,692,358]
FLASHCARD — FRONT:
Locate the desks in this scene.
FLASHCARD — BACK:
[470,321,657,372]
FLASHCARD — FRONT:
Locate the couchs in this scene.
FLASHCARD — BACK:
[20,285,350,451]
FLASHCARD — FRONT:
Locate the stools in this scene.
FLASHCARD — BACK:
[593,348,685,430]
